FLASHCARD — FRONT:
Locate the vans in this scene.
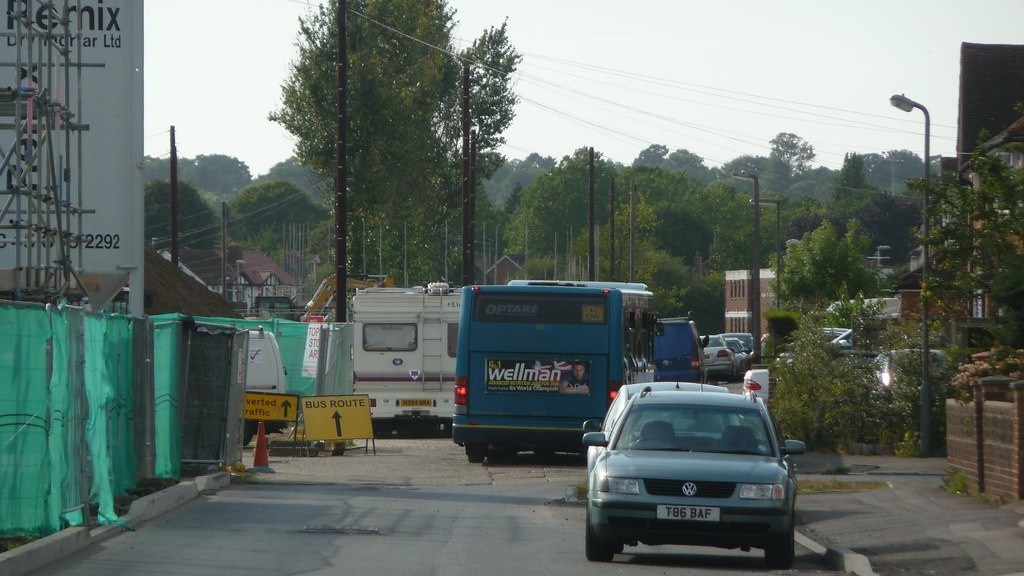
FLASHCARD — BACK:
[649,311,710,387]
[237,327,288,448]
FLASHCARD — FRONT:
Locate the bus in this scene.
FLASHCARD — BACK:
[451,281,667,465]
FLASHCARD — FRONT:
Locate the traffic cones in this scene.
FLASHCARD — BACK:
[244,419,275,474]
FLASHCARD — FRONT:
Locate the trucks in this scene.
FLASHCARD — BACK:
[350,284,466,439]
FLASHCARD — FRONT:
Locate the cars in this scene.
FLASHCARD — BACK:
[873,349,947,394]
[717,328,859,377]
[581,381,731,475]
[696,335,736,382]
[743,351,882,401]
[583,386,809,570]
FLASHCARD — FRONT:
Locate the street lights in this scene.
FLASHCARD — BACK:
[889,93,932,455]
[731,168,762,365]
[747,197,781,313]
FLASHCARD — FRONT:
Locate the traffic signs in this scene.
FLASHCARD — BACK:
[244,392,301,423]
[301,393,375,440]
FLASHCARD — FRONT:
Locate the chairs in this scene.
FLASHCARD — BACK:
[640,420,676,443]
[716,425,759,451]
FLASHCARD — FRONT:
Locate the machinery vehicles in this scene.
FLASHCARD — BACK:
[296,269,397,321]
